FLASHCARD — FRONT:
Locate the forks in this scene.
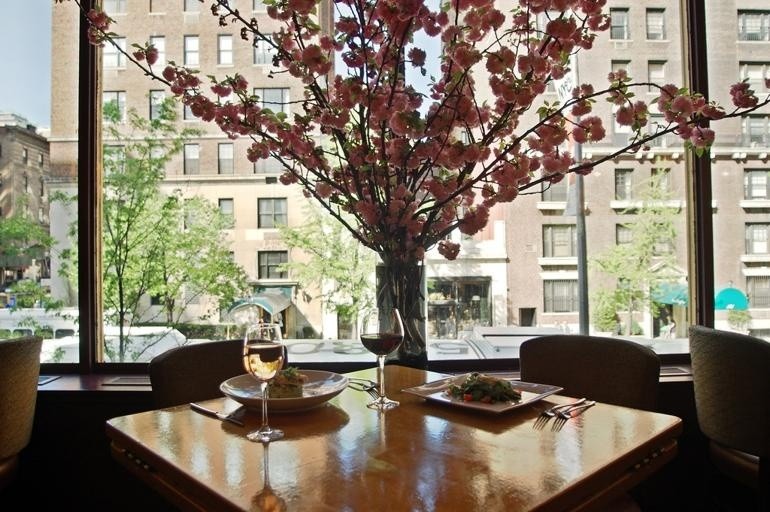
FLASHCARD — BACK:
[531,397,595,419]
[347,376,380,391]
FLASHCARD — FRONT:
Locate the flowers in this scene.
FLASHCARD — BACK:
[54,0,770,345]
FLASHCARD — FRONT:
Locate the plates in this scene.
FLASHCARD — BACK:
[217,369,349,413]
[223,402,350,442]
[401,371,563,416]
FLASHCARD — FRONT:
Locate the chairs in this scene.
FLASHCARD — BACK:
[689,322,770,492]
[148,339,289,409]
[0,334,43,495]
[518,335,661,412]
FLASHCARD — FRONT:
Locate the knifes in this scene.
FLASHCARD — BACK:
[189,403,245,428]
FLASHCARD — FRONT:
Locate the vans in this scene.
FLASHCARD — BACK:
[103,327,209,365]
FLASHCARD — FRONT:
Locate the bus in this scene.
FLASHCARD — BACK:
[0,305,131,344]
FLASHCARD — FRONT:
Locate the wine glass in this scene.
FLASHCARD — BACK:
[250,443,289,512]
[242,325,285,444]
[361,307,405,410]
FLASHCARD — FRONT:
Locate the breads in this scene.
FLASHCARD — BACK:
[270,373,303,397]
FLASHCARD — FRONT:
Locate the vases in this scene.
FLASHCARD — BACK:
[375,265,429,372]
